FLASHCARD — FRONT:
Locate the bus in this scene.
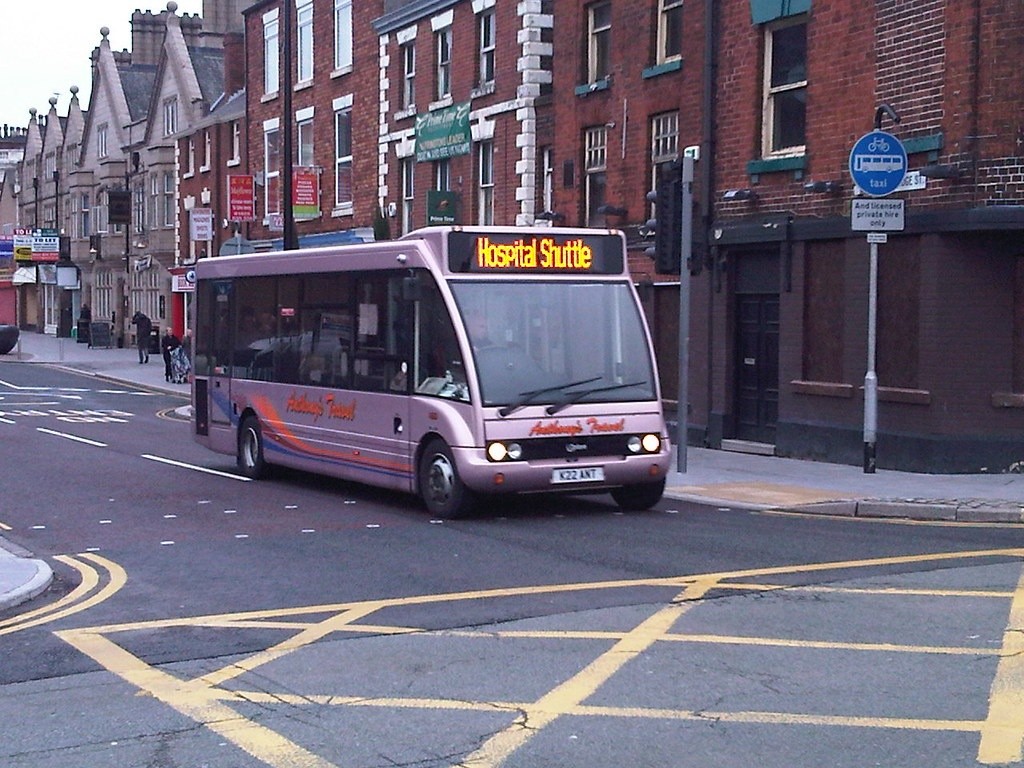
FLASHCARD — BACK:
[188,225,671,520]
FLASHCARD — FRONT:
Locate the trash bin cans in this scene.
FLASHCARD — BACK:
[77,321,88,343]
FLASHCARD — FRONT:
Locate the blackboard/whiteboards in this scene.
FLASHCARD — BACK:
[88,322,113,346]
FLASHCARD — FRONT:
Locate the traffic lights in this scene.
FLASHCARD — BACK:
[644,173,681,276]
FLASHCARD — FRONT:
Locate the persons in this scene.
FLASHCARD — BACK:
[442,313,507,358]
[131,311,151,364]
[78,303,91,320]
[162,327,181,382]
[182,330,194,383]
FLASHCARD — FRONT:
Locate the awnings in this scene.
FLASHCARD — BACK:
[12,265,37,286]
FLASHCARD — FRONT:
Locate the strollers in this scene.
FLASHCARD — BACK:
[169,346,191,384]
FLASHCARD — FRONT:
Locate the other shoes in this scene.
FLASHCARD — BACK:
[145,355,148,363]
[140,361,143,364]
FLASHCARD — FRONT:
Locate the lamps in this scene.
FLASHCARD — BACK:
[720,189,758,201]
[596,205,627,216]
[536,212,564,221]
[919,165,970,180]
[803,182,845,194]
